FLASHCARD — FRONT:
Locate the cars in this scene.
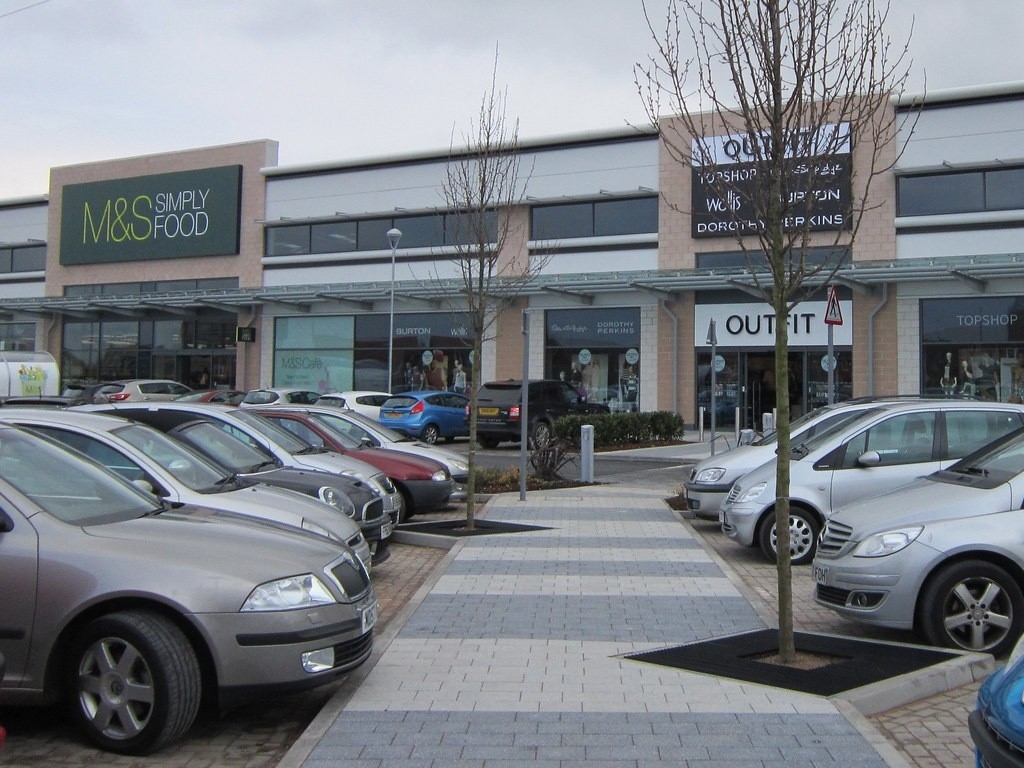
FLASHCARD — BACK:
[683,393,986,522]
[247,409,453,522]
[812,426,1024,659]
[260,405,469,499]
[238,387,320,409]
[720,401,1024,564]
[315,390,393,421]
[64,402,394,565]
[174,403,401,529]
[375,391,469,445]
[1,420,379,755]
[94,379,190,401]
[172,389,245,405]
[1,405,373,576]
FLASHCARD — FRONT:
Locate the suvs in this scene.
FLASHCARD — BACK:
[463,378,610,452]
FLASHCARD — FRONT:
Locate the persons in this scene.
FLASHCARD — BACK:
[974,355,999,400]
[938,352,957,395]
[569,361,582,390]
[451,360,460,386]
[580,359,599,401]
[403,362,413,391]
[625,366,639,402]
[559,370,566,384]
[619,357,635,399]
[452,364,467,395]
[1008,349,1024,403]
[423,360,447,392]
[409,366,424,392]
[960,361,978,399]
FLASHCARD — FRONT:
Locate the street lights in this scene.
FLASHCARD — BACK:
[386,229,403,394]
